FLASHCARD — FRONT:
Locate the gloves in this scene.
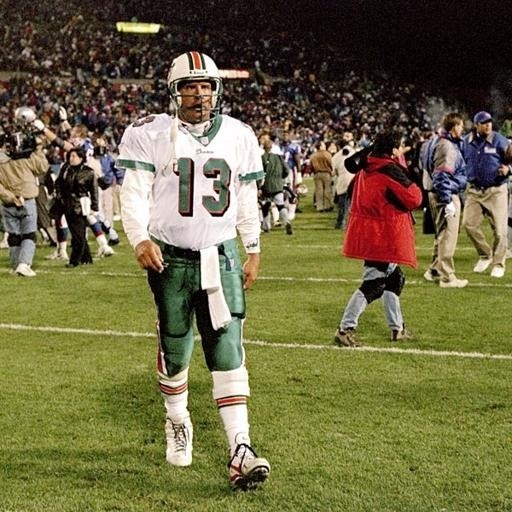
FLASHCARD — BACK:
[445,202,455,219]
[59,107,67,120]
[31,120,45,131]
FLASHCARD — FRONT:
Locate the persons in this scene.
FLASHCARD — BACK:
[0,0,511,280]
[331,126,423,348]
[417,111,470,290]
[113,49,272,487]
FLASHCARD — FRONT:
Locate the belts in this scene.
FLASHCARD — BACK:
[165,243,231,271]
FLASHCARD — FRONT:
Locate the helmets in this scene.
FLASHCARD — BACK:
[167,52,223,124]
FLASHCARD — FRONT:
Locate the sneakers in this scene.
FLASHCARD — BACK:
[491,267,505,278]
[424,267,440,281]
[165,413,193,467]
[15,263,35,277]
[227,443,270,491]
[334,327,362,347]
[473,258,492,272]
[390,323,413,342]
[45,252,69,261]
[440,276,469,288]
[97,246,115,258]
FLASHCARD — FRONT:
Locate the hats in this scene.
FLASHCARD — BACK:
[474,112,494,123]
